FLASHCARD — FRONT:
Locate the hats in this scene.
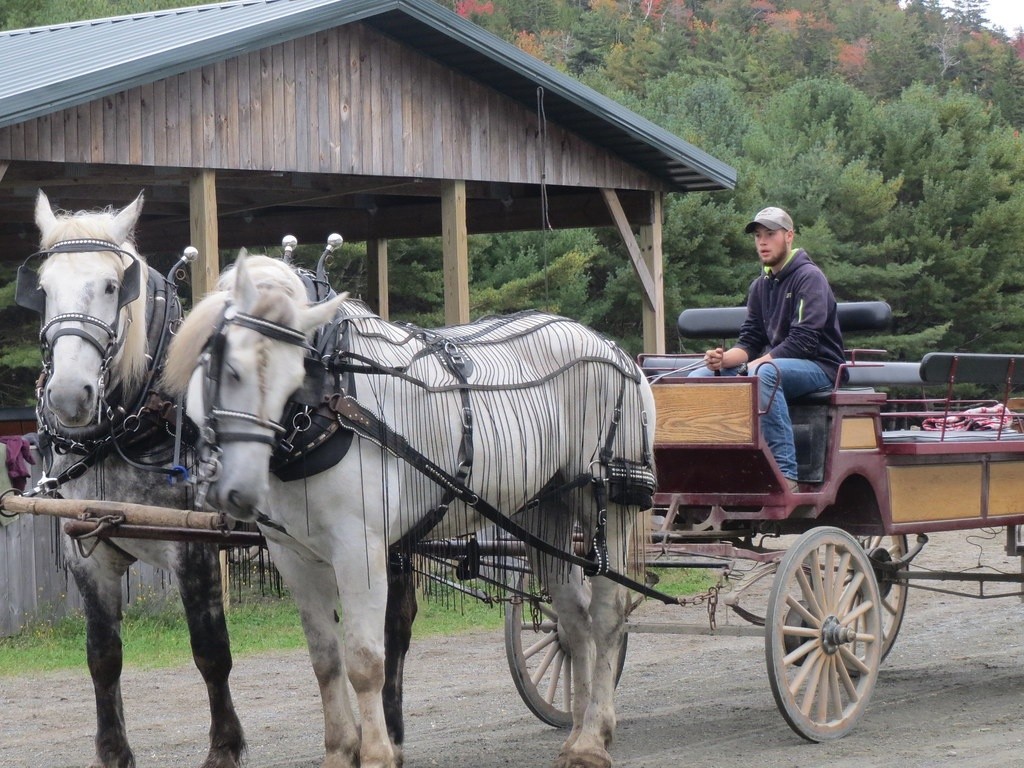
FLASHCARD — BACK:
[745,207,792,234]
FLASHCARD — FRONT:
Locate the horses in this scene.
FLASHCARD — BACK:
[157,245,657,767]
[35,185,433,768]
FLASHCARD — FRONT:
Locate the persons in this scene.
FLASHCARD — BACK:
[689,206,848,494]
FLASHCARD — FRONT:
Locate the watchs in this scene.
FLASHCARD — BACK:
[737,362,748,376]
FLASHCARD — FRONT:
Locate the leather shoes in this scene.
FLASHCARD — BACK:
[783,478,799,494]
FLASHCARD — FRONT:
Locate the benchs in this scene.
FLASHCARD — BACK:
[642,301,892,485]
[846,351,1024,443]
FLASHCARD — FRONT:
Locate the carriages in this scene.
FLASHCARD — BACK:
[0,186,1023,768]
